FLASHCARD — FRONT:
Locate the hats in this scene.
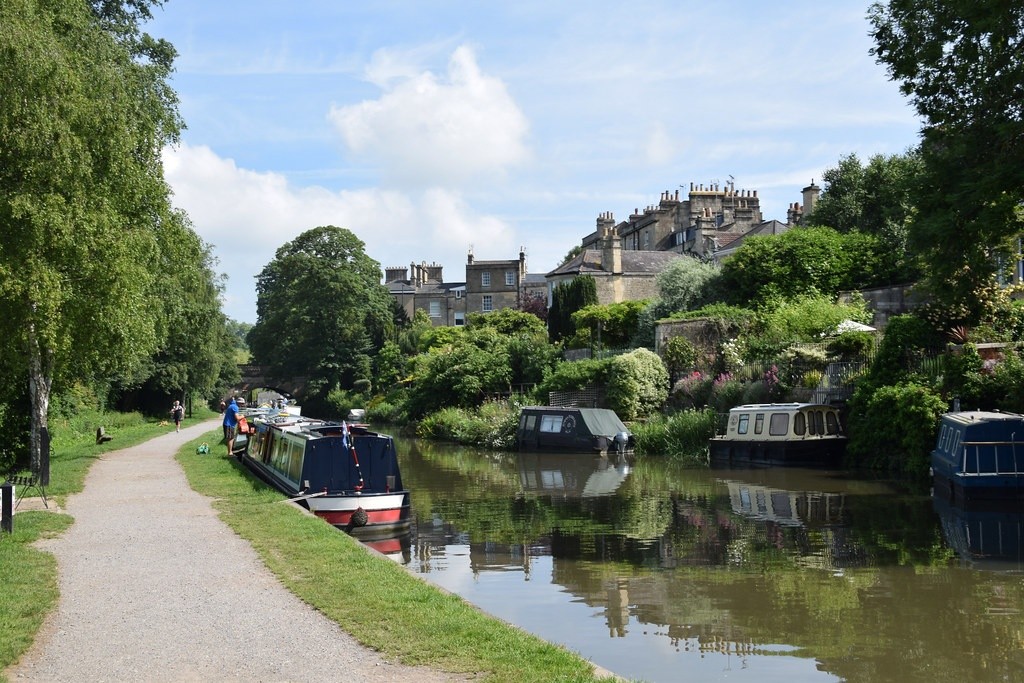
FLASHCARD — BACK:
[237,398,245,403]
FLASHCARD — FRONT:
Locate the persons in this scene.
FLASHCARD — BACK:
[223,396,245,456]
[220,399,226,414]
[172,401,184,432]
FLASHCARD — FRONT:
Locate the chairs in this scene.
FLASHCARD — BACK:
[4,426,51,510]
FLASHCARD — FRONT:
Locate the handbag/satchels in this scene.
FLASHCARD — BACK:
[238,418,249,435]
[197,443,210,455]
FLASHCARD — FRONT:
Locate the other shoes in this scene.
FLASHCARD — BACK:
[176,429,180,432]
[228,455,237,458]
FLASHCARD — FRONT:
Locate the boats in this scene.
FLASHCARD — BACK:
[929,407,1024,503]
[704,401,849,466]
[516,405,636,455]
[227,398,414,531]
